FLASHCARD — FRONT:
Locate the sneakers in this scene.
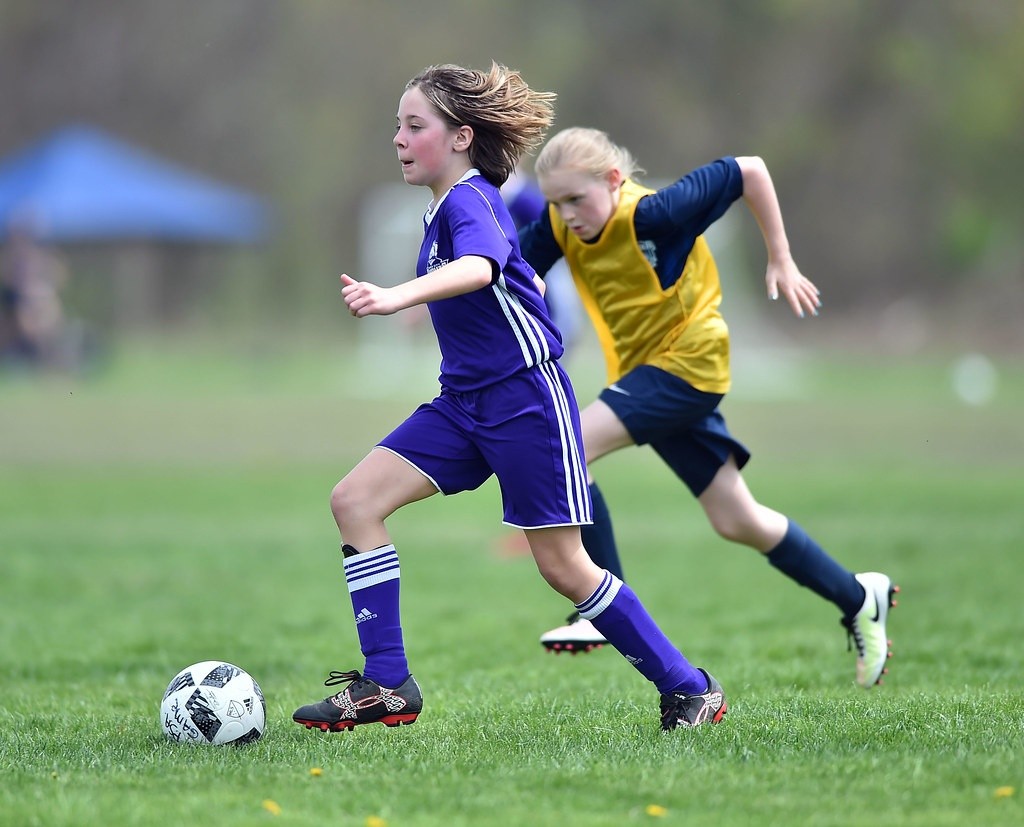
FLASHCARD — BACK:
[842,572,899,688]
[654,666,727,729]
[293,668,423,732]
[541,611,610,654]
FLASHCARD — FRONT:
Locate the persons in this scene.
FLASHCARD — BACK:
[290,61,728,736]
[516,123,901,693]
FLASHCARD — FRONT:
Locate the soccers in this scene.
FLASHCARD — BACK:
[159,661,267,749]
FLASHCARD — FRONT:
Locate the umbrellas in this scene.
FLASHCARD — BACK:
[0,117,278,250]
